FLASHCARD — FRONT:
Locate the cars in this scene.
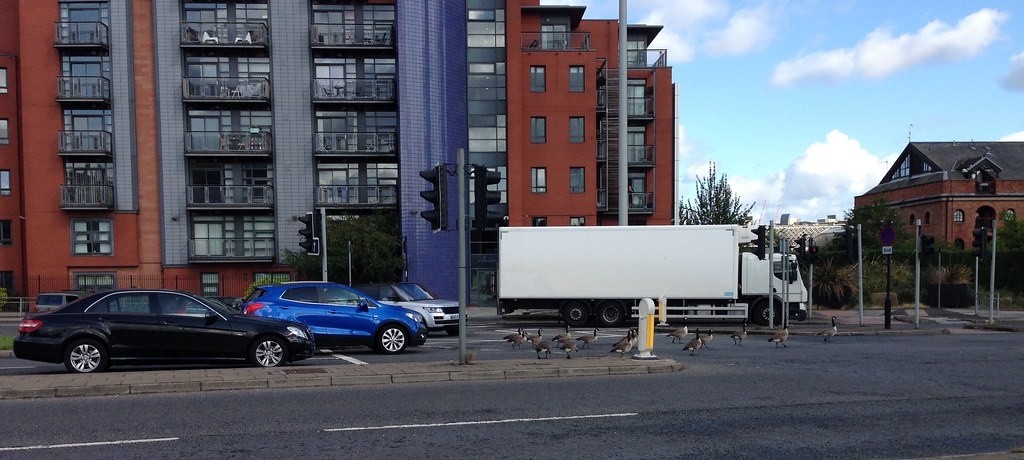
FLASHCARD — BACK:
[185,296,244,314]
[12,287,317,373]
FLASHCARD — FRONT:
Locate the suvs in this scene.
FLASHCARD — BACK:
[232,279,429,355]
[322,281,469,337]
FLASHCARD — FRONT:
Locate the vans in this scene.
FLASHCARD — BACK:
[35,292,81,315]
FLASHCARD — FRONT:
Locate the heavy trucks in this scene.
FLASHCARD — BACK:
[495,223,809,327]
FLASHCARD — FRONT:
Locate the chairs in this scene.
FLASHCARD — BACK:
[238,136,247,150]
[219,137,229,151]
[234,33,252,45]
[201,31,220,45]
[230,85,243,99]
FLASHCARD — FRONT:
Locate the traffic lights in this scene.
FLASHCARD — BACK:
[474,164,501,228]
[419,162,441,234]
[973,228,986,259]
[922,234,934,256]
[297,211,316,253]
[810,246,817,254]
[794,237,805,259]
[750,225,765,260]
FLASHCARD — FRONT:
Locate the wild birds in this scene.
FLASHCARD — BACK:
[730,322,748,346]
[534,341,551,359]
[666,316,689,344]
[576,328,601,348]
[682,327,702,356]
[608,329,635,357]
[527,328,544,348]
[613,328,639,354]
[767,317,790,348]
[702,328,715,349]
[559,338,588,355]
[507,331,528,350]
[814,316,838,342]
[503,327,523,347]
[551,325,572,348]
